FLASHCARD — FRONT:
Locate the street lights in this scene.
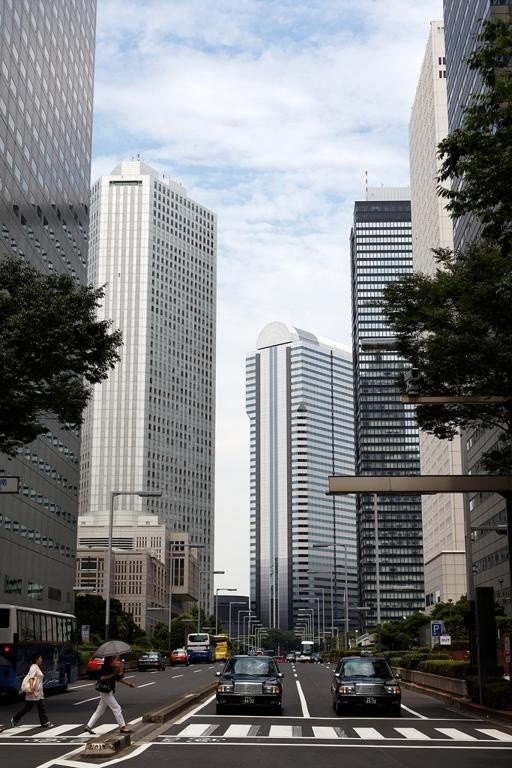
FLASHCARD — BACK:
[293,488,382,653]
[103,485,164,647]
[166,542,206,653]
[197,569,270,656]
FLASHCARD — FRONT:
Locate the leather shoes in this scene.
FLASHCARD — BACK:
[84,727,96,734]
[120,728,135,733]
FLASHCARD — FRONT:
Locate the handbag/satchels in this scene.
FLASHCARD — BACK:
[95,680,111,693]
[21,675,38,693]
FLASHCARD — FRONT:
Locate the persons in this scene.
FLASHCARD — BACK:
[83,655,136,734]
[9,654,55,729]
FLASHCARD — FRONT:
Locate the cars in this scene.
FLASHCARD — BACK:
[330,654,403,714]
[138,651,168,672]
[214,654,286,709]
[273,638,324,664]
[170,648,192,667]
[86,654,125,679]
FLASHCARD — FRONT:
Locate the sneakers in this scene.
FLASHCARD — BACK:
[42,722,54,728]
[11,717,20,725]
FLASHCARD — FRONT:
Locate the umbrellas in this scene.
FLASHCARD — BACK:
[91,640,132,672]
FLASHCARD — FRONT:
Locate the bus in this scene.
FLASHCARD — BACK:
[0,602,79,698]
[212,633,232,661]
[187,632,218,663]
